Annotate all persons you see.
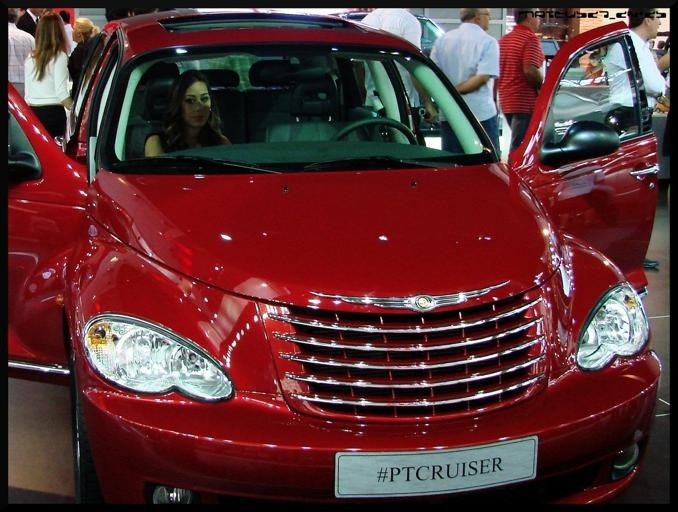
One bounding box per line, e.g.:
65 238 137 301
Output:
657 50 670 225
580 7 671 135
492 7 544 167
426 6 501 159
142 68 232 156
7 9 99 145
359 8 438 148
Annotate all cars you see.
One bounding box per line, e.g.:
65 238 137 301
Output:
332 11 446 56
540 40 563 68
8 7 662 508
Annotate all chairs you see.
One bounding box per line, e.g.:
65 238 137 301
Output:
124 58 361 147
